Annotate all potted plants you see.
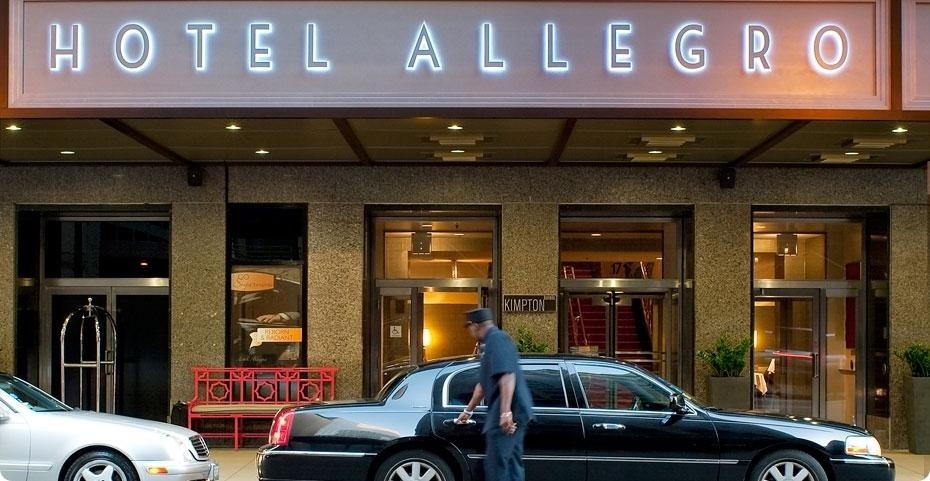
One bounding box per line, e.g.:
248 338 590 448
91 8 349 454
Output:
698 332 755 412
892 341 930 456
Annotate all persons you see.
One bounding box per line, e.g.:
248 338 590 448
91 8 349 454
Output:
455 307 539 481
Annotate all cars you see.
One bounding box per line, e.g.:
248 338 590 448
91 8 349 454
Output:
253 344 901 480
751 346 890 423
0 369 224 480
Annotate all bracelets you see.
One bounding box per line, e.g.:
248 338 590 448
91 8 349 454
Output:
463 408 474 416
499 411 513 418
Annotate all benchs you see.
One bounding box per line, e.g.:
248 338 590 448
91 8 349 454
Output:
187 367 337 453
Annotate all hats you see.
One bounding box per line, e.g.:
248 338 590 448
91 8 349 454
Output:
463 307 493 328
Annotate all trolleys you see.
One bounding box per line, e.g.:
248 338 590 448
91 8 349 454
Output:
58 294 121 415
